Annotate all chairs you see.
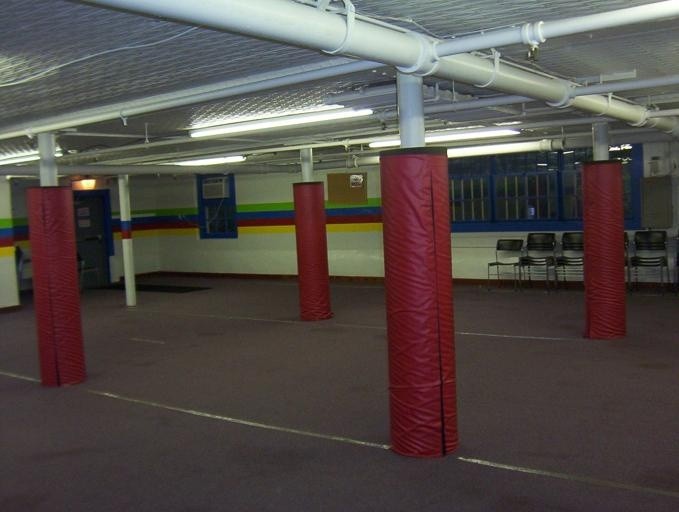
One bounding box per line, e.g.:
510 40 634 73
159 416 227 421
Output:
486 231 672 297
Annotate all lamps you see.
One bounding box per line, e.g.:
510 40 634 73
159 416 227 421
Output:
0 102 521 167
79 175 96 190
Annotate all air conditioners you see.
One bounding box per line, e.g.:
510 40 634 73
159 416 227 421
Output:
203 176 230 199
